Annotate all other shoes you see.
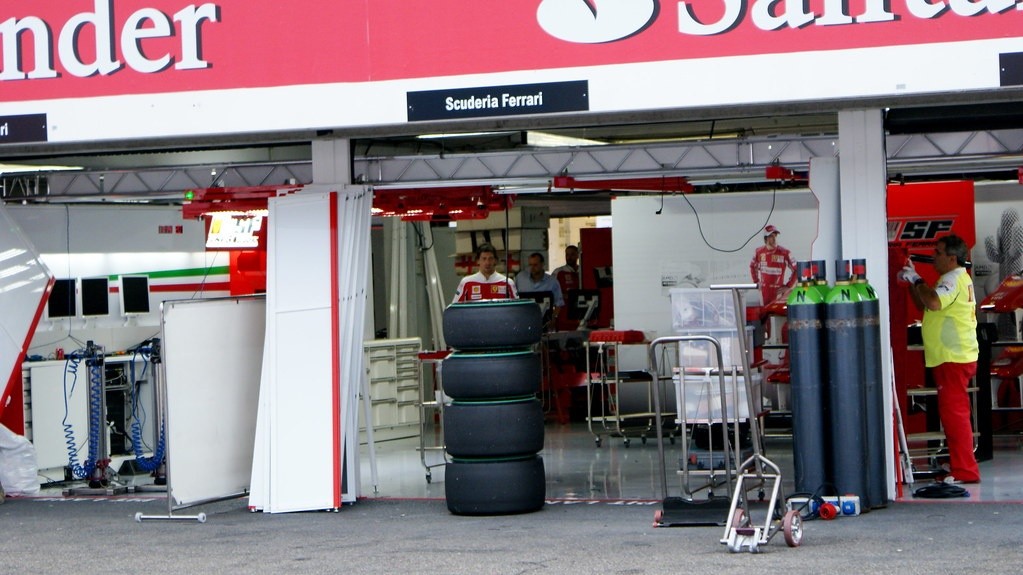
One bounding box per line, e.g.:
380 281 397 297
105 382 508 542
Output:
936 475 948 483
944 475 966 484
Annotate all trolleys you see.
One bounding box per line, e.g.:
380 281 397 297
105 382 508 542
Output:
583 328 678 449
416 350 452 487
708 282 805 553
644 335 775 527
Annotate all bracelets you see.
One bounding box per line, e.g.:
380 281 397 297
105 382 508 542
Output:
914 278 926 288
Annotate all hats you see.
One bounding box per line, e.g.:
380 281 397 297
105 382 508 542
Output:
764 225 780 237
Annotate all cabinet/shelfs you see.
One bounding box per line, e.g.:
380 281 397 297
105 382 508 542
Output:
356 337 425 445
21 354 166 490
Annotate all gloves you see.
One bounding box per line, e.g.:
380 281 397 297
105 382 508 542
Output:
900 259 923 286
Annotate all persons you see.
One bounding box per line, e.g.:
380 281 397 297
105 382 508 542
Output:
452 243 519 302
514 245 565 327
749 224 797 306
902 234 980 483
551 245 579 298
